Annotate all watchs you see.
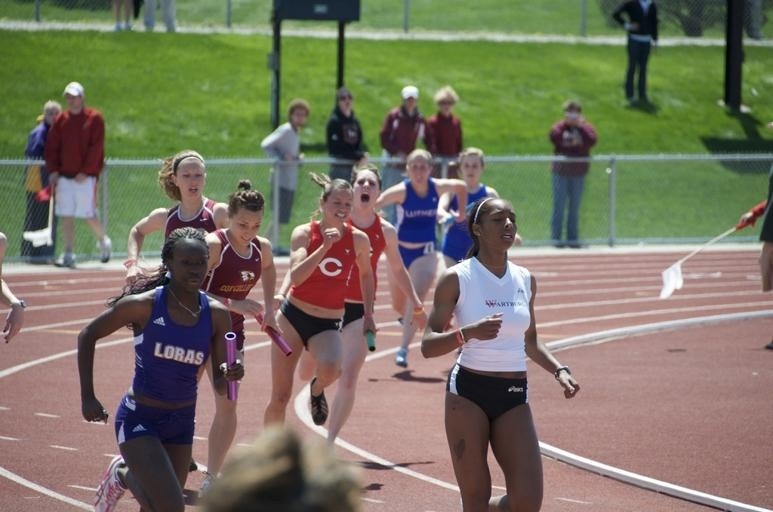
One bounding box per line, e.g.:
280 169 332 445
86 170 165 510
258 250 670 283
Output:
10 300 27 309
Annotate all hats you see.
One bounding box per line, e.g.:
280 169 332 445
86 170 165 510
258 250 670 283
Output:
62 82 84 97
399 86 420 100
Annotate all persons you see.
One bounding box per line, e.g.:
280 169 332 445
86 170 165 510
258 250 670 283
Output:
375 143 472 370
113 2 176 32
260 99 311 257
437 147 524 269
612 0 659 110
74 223 247 511
549 99 596 248
415 194 582 511
24 82 112 269
0 228 31 351
196 177 288 495
260 177 383 433
184 421 376 510
121 142 234 299
735 161 773 354
380 85 464 227
326 87 371 186
268 158 429 450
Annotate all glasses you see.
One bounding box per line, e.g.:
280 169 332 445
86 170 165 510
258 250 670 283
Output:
341 95 353 100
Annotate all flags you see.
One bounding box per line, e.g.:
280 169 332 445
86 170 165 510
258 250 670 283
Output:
658 257 685 301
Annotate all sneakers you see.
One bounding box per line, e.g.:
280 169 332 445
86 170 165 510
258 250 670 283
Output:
97 235 112 262
394 351 406 367
94 455 126 512
309 377 329 426
54 252 78 266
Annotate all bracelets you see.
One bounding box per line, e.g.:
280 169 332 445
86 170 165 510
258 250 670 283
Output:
459 328 466 344
554 365 572 380
362 311 375 321
124 258 138 267
456 330 463 345
412 306 427 316
